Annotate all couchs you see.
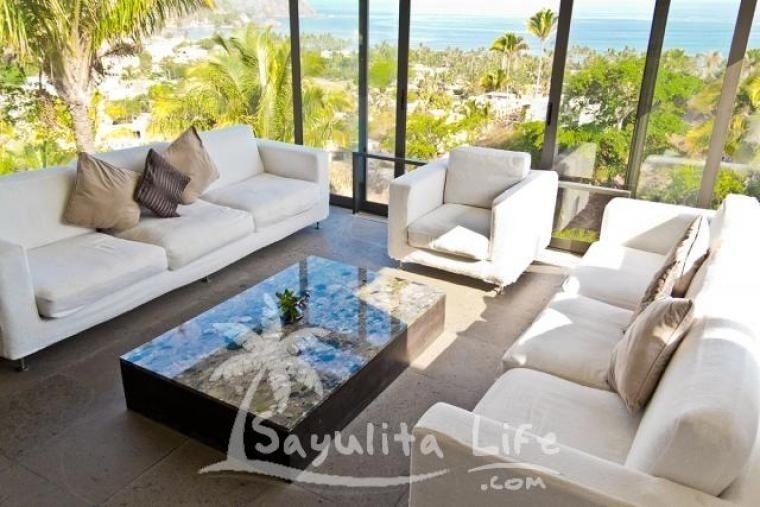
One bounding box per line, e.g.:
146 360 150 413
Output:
1 124 329 370
387 143 559 288
407 192 760 507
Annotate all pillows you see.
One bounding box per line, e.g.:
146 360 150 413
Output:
133 147 192 217
160 125 220 206
622 260 681 335
604 295 695 414
663 213 710 296
61 152 142 229
